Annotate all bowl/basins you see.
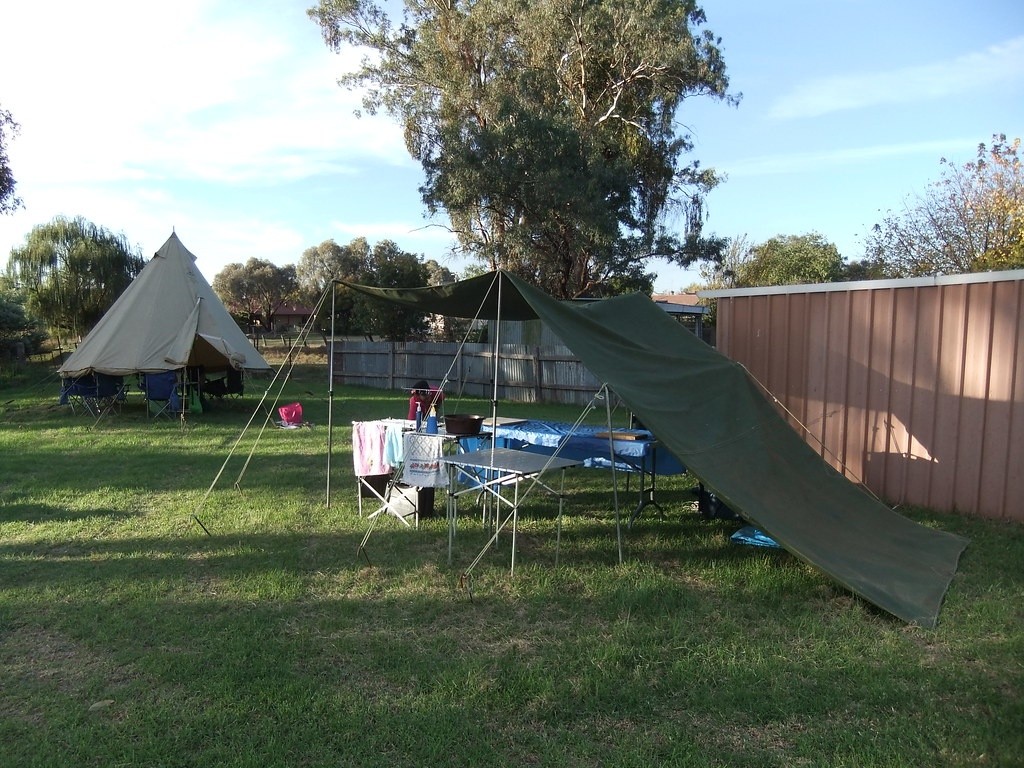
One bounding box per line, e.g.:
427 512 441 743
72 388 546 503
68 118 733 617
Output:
444 413 485 436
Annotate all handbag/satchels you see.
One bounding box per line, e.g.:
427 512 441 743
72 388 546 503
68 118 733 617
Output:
277 402 303 424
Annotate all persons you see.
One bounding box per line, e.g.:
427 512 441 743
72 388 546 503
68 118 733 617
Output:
406 381 445 421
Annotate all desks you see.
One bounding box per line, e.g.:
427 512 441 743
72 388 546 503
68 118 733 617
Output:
351 418 494 532
456 417 666 531
432 447 583 579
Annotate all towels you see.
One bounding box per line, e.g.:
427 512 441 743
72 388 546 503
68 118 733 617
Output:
402 430 451 488
382 420 412 472
350 420 394 477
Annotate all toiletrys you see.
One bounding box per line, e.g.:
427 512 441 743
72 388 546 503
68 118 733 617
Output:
426 402 441 435
413 400 422 434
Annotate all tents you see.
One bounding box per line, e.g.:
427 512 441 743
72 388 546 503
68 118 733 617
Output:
55 226 278 435
202 266 972 632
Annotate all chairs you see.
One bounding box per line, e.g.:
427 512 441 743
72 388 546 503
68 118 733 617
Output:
61 364 246 424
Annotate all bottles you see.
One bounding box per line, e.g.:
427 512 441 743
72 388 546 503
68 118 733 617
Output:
426 403 439 435
415 401 423 433
